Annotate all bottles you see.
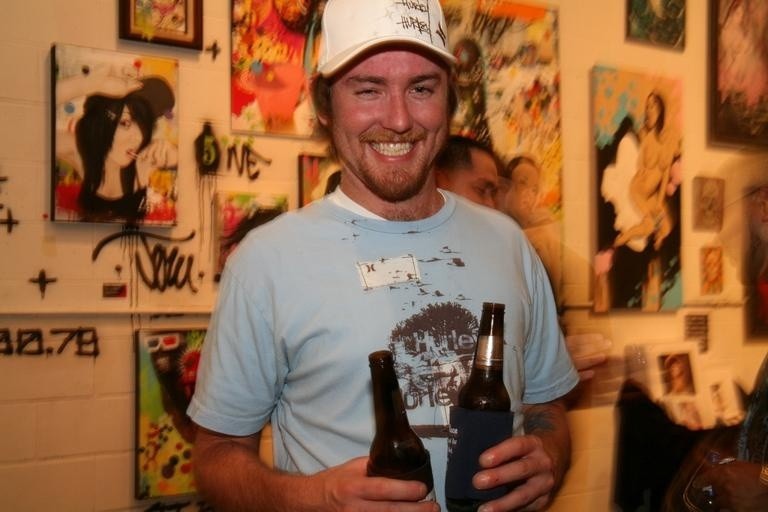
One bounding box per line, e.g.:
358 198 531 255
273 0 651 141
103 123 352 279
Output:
446 302 523 511
368 349 436 507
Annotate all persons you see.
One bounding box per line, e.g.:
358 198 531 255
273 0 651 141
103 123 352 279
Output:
435 136 498 209
56 59 177 228
595 88 676 276
505 155 552 231
187 0 579 512
145 332 188 415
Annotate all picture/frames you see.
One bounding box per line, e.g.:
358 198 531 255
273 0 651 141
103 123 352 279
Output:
119 0 203 50
707 0 768 150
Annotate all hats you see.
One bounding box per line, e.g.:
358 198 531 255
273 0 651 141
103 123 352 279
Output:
316 2 460 78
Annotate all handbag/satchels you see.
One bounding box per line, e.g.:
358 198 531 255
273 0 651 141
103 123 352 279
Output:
661 439 767 511
